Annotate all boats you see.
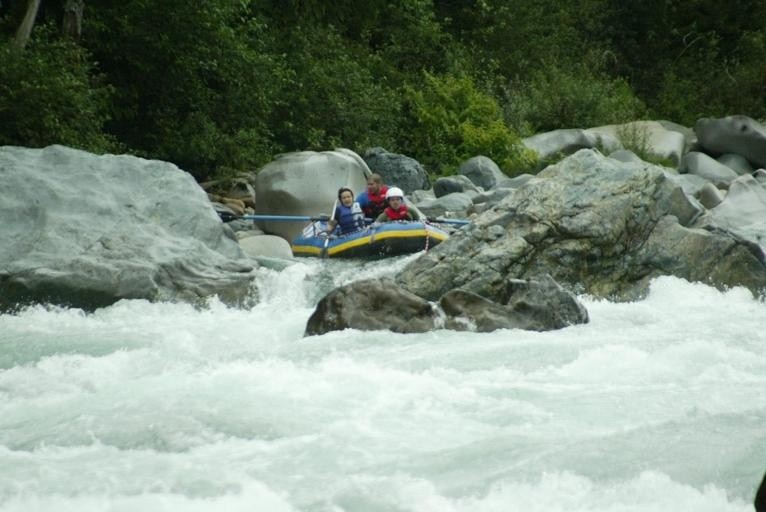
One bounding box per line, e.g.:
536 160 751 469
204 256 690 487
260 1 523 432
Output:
294 212 451 261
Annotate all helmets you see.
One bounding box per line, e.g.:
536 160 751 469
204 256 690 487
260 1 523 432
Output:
384 187 404 202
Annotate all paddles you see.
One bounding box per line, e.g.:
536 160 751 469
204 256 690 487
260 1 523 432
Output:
216 210 330 223
319 198 338 259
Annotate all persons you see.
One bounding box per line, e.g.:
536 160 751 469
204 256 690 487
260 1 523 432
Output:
353 173 391 220
373 185 419 225
326 187 368 238
